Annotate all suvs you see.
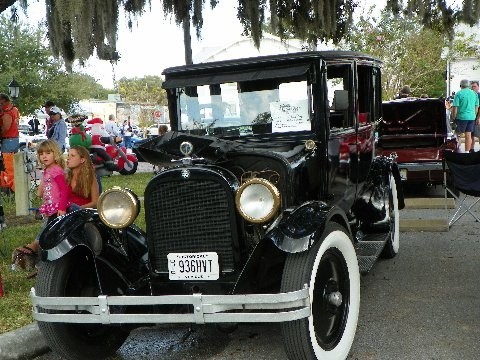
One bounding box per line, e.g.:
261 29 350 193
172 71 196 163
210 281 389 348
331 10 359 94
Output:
384 84 456 185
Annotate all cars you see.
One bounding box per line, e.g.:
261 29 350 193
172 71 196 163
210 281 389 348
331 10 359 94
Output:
28 51 404 360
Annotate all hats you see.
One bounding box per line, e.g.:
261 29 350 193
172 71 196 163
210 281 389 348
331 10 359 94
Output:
43 101 54 107
49 107 61 114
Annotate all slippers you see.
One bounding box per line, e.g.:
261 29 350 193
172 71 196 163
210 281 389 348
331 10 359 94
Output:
16 246 38 255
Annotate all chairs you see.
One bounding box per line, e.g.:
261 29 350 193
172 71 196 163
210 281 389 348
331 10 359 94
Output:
0 152 16 204
441 145 480 229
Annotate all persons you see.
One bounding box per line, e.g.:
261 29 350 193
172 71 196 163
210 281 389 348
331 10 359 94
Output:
43 101 67 154
420 94 429 98
106 114 118 142
181 109 188 126
445 79 480 153
393 84 410 100
38 140 69 217
119 119 132 149
65 112 122 171
28 116 40 135
159 126 168 135
15 145 100 256
0 93 20 154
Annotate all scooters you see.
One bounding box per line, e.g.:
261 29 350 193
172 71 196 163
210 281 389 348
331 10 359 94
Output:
90 136 138 178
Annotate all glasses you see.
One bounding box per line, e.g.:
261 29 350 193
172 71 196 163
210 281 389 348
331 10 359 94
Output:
48 112 56 116
